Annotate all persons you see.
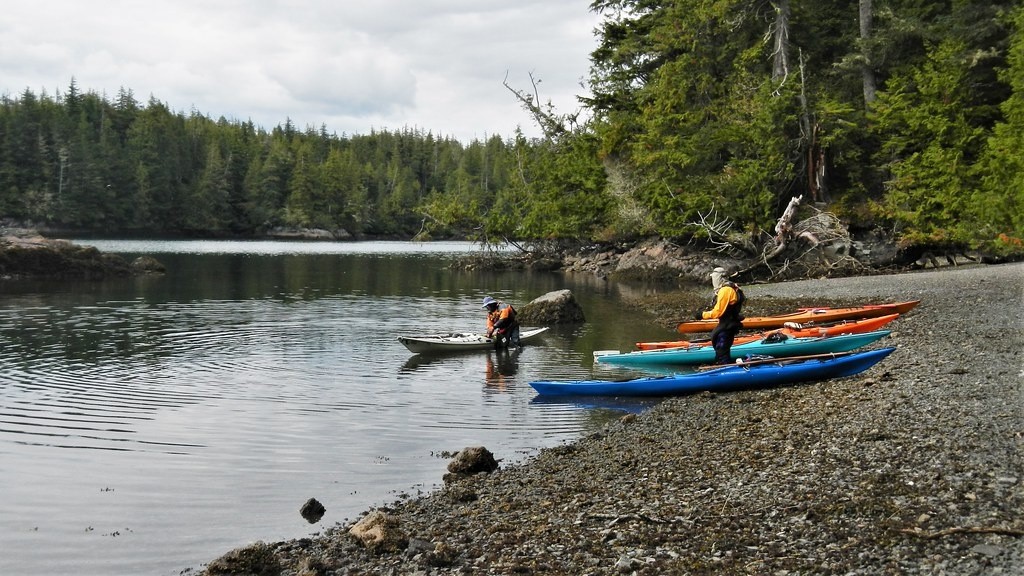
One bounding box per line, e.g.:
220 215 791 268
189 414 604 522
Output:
695 268 745 365
482 296 520 351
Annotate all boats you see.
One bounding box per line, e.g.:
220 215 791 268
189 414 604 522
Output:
677 298 921 336
529 346 898 400
397 327 551 354
636 312 900 350
593 328 891 373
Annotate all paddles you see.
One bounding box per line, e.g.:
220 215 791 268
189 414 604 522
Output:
797 304 896 311
699 351 851 370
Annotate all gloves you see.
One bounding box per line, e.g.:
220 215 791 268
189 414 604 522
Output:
695 312 703 320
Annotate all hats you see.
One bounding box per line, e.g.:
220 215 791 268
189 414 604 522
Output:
482 296 497 308
